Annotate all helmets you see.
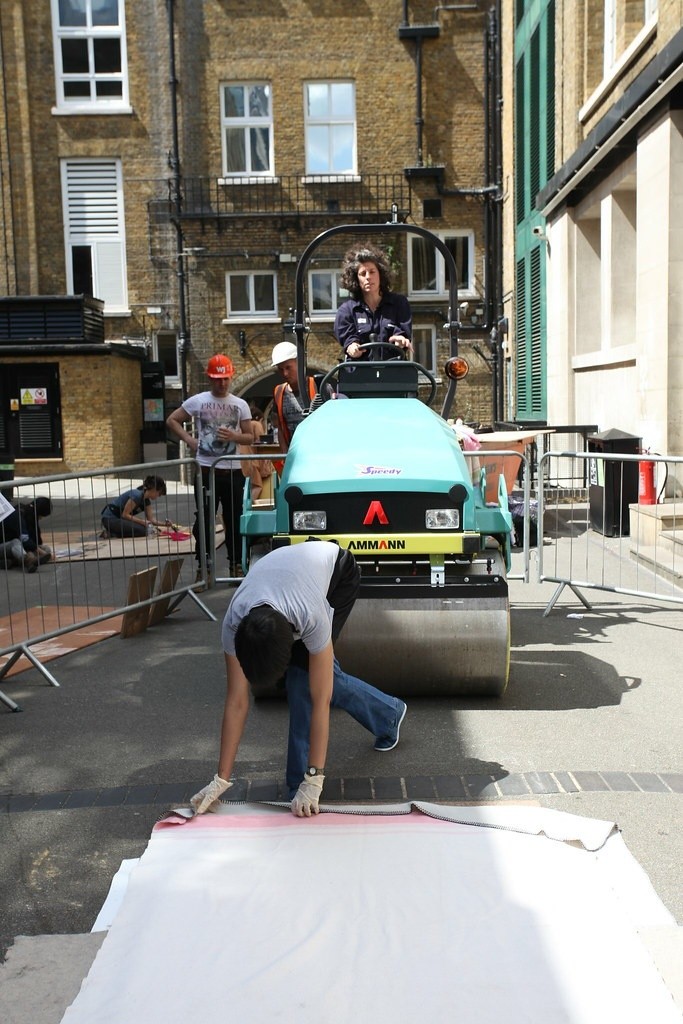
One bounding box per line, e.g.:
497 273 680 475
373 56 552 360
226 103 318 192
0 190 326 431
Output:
207 354 235 379
271 342 297 367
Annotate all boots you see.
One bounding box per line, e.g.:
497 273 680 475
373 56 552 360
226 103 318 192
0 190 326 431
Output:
25 552 52 572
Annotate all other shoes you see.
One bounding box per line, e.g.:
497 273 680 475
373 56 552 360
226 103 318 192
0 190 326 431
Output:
230 566 244 587
99 530 110 539
193 571 210 592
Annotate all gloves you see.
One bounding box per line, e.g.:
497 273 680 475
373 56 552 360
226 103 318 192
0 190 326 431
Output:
291 773 326 817
190 774 233 814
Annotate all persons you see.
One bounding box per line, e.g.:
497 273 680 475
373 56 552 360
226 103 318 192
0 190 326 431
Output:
101 476 171 539
271 341 319 452
166 354 255 590
334 247 414 362
0 497 50 573
190 541 407 819
240 408 265 505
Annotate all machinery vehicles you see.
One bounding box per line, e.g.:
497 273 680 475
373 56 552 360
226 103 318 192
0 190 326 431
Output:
240 221 513 713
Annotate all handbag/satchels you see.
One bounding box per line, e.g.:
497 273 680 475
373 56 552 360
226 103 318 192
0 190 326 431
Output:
259 457 274 478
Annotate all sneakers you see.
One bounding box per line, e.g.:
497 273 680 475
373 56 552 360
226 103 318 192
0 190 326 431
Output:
374 698 407 751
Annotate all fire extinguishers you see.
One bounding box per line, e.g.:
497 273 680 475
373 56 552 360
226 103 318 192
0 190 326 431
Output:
635 446 661 505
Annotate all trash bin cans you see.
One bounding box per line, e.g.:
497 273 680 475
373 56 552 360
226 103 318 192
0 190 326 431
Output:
586 427 643 538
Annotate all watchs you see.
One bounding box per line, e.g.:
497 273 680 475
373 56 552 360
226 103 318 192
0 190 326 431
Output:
306 766 324 776
